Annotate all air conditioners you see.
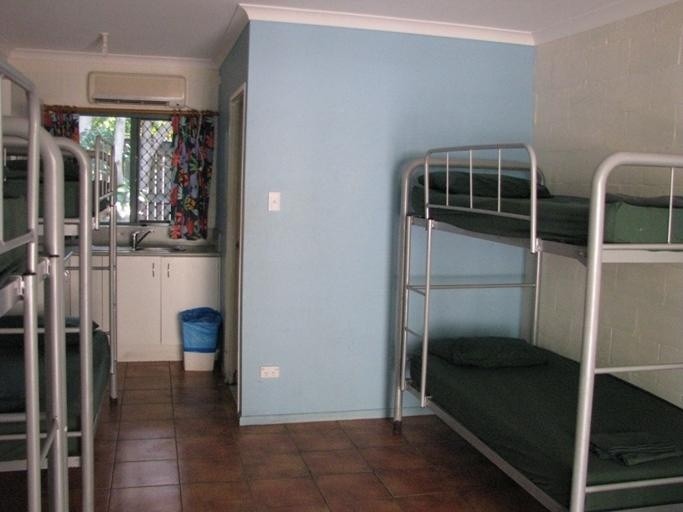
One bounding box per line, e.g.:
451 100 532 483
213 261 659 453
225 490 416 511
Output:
87 71 186 107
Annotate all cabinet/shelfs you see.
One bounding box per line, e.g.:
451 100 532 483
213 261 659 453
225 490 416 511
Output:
5 256 221 362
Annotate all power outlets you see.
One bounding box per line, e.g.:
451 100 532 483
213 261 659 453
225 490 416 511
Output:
259 366 279 379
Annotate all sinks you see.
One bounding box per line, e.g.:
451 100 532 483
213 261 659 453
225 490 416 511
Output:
92 244 132 253
136 246 185 252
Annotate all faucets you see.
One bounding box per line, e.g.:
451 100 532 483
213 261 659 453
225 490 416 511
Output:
131 229 153 251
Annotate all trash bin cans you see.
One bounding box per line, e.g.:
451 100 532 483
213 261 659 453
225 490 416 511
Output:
177 307 222 372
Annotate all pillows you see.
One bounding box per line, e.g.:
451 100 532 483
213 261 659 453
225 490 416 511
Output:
418 170 552 197
422 337 549 369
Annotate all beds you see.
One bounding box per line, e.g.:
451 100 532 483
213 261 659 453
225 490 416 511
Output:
388 143 682 512
